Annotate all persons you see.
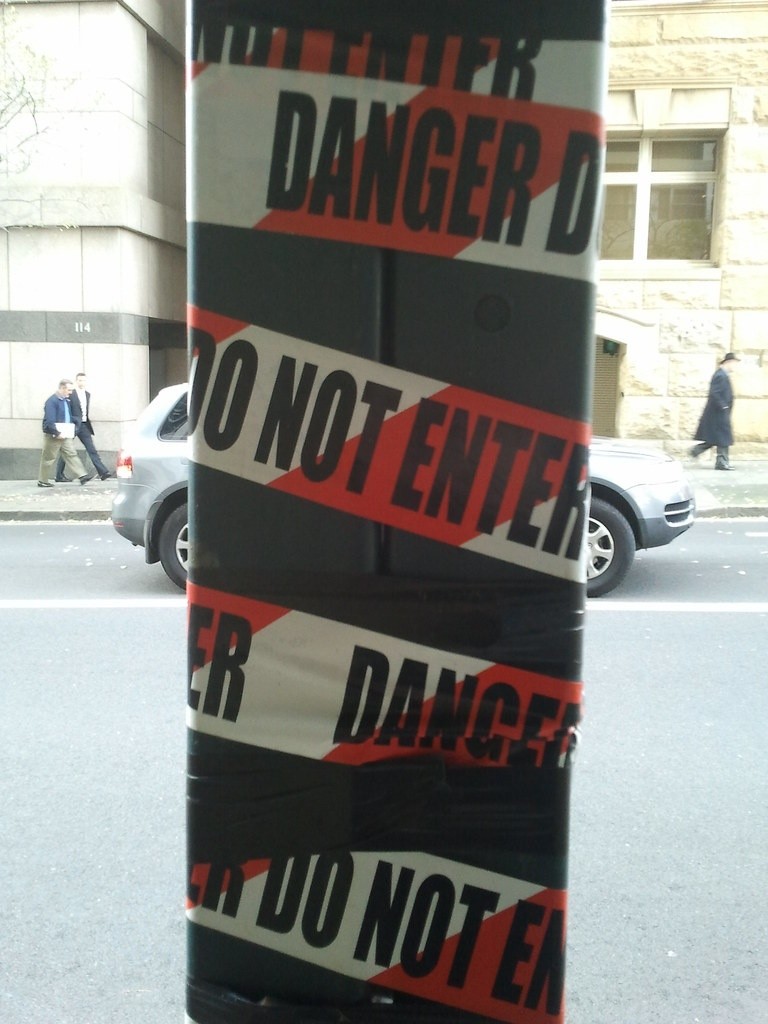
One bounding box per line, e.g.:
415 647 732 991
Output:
55 373 117 482
36 380 98 487
690 352 742 470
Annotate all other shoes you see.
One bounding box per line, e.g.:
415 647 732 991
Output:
55 476 72 482
38 481 53 487
80 476 94 485
715 464 736 470
101 470 116 481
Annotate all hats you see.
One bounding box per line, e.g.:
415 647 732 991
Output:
719 353 741 364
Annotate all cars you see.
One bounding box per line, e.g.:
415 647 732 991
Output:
112 378 696 596
